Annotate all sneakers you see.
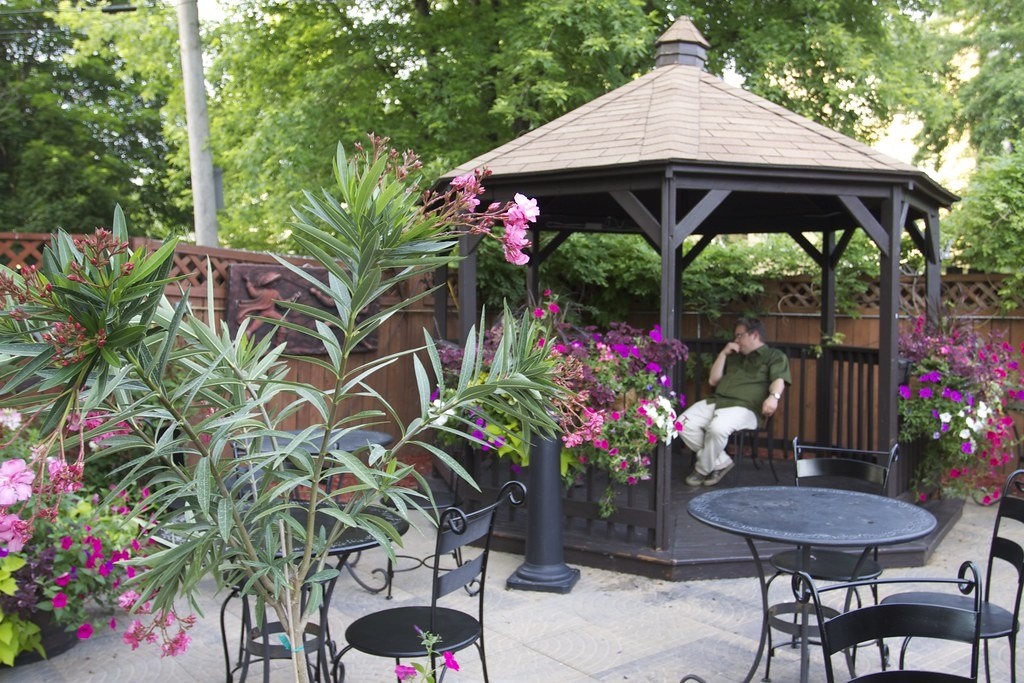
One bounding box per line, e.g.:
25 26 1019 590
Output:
704 463 735 486
686 470 704 486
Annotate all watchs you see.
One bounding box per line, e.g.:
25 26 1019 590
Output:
769 393 781 399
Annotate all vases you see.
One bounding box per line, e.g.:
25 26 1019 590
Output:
6 594 80 667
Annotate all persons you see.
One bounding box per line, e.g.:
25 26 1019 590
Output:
676 317 791 486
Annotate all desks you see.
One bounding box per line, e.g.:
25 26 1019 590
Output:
197 496 410 683
234 427 393 504
679 485 936 683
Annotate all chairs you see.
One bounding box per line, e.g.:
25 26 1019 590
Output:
689 379 781 483
380 403 481 600
234 442 339 509
765 437 1024 683
333 481 531 683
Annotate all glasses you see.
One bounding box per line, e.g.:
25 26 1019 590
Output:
736 331 749 337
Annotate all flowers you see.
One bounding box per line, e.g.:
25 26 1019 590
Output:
0 408 194 670
